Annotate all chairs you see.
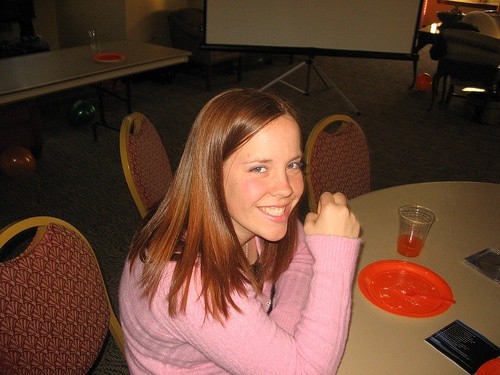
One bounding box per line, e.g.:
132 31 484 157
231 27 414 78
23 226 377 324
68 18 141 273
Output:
440 29 500 102
120 111 173 219
168 8 242 91
303 114 370 215
6 34 74 157
0 216 130 375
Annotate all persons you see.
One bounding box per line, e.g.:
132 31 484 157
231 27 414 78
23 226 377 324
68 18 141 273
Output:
120 88 362 375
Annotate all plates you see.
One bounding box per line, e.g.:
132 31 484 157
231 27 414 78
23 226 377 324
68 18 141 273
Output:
358 259 454 318
90 52 127 63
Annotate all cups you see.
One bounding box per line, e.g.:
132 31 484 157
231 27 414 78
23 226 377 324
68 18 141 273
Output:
396 205 435 258
87 30 100 56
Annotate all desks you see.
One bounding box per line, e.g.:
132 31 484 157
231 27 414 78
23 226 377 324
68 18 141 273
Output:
336 182 500 375
0 40 190 142
408 23 447 94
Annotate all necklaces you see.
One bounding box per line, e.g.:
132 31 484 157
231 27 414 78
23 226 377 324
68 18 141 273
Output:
245 242 248 259
